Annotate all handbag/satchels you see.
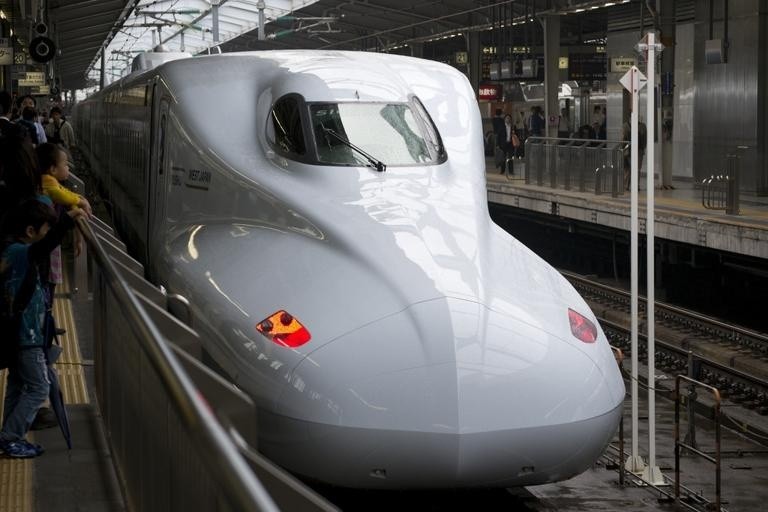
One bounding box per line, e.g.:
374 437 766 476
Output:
511 135 520 147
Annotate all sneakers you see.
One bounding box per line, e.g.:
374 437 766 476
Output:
0 438 45 458
28 407 59 430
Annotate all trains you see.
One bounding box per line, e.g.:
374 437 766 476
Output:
66 43 628 497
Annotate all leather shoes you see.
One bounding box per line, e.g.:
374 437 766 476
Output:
54 328 65 335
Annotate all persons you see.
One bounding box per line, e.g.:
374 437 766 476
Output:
0 86 92 460
491 104 646 194
